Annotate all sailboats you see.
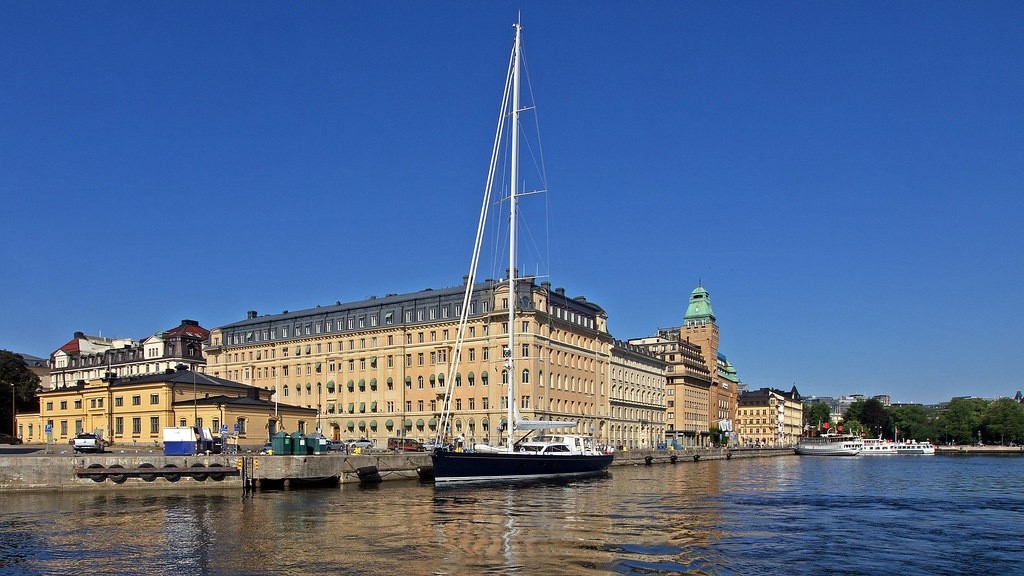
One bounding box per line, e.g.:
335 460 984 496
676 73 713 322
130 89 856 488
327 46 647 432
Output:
427 7 612 486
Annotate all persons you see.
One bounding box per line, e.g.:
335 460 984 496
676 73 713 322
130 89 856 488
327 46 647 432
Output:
458 434 465 452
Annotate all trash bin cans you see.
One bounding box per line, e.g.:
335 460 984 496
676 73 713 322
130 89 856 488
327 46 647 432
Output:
304 432 328 454
271 431 292 455
162 426 204 455
290 431 308 454
197 426 214 453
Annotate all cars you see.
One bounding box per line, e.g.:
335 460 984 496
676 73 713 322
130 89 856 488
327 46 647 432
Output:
423 442 440 451
326 440 345 453
345 439 374 449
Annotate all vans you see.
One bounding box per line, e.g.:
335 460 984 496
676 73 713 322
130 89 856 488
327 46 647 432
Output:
387 438 423 452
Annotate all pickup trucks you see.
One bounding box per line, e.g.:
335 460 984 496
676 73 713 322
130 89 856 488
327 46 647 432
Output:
69 432 106 453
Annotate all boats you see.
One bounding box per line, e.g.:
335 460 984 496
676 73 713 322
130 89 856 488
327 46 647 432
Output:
859 437 935 455
787 430 862 455
